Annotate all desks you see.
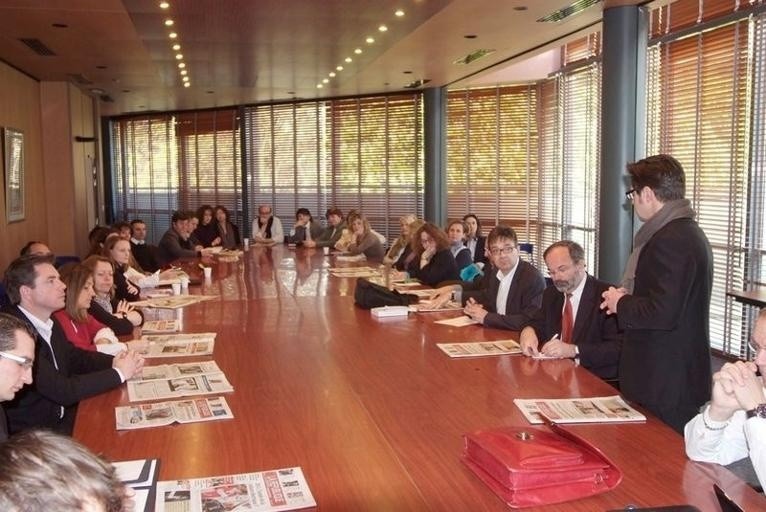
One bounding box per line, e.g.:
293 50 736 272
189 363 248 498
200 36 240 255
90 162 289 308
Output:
71 244 766 512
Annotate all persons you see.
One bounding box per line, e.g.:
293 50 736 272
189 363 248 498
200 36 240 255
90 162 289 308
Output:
601 154 714 437
684 305 766 498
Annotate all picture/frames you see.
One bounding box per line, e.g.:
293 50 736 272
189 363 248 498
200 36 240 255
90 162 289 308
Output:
2 126 25 225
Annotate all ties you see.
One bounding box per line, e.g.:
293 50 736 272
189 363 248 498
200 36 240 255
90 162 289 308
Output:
561 293 574 345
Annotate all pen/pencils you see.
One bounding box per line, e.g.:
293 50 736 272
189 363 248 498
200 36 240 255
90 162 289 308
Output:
541 333 559 355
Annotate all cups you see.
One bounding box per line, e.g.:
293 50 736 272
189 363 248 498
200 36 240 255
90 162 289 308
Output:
171 266 213 295
323 246 330 255
243 237 251 246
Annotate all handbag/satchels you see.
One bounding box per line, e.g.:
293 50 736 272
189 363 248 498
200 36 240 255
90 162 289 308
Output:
459 410 624 509
353 277 420 310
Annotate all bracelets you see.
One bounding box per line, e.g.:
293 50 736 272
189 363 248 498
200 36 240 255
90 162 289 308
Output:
701 402 731 432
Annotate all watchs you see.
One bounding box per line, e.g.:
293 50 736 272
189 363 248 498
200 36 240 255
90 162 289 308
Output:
740 404 765 419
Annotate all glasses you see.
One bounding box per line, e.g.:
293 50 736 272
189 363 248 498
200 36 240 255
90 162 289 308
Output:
748 338 766 353
0 350 33 370
625 187 638 200
490 246 514 256
